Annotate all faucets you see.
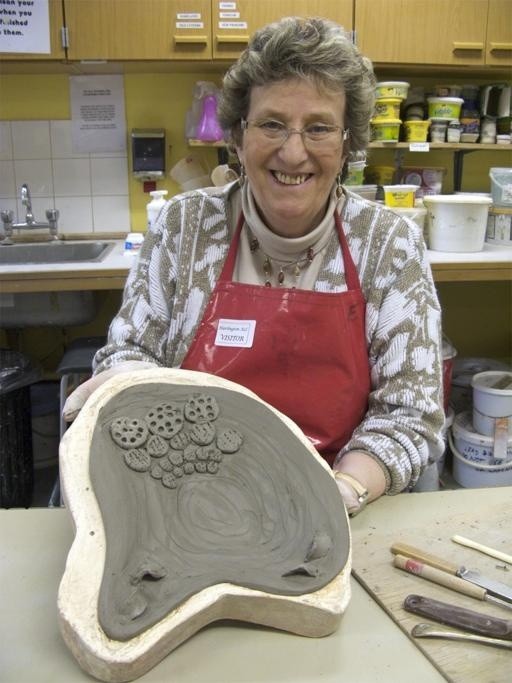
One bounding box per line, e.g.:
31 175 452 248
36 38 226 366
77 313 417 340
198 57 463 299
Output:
21 182 34 223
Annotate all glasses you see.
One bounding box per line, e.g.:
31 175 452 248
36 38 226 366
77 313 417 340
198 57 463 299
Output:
238 115 350 152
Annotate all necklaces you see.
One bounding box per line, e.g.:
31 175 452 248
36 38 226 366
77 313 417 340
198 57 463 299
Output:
246 232 315 289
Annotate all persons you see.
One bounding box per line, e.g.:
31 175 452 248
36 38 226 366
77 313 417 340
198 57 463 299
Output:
60 17 447 515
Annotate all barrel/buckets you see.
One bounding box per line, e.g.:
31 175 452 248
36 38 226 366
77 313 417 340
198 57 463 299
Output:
489 167 512 206
438 339 458 417
448 355 512 417
471 370 512 438
449 411 512 489
390 207 427 231
423 195 495 252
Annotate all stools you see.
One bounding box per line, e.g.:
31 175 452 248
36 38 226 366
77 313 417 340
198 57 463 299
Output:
49 337 108 512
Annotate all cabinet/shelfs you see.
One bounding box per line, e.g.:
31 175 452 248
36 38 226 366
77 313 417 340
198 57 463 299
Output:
63 0 354 62
354 0 512 72
0 0 64 63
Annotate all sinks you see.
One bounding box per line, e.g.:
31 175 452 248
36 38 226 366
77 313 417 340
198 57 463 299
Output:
0 240 116 263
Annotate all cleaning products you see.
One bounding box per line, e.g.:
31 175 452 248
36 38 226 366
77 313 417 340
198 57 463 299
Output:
147 191 168 225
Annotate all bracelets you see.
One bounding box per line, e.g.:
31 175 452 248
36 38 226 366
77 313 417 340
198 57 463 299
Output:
335 470 369 516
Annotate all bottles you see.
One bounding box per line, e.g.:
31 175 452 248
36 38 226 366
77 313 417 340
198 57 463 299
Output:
125 232 145 256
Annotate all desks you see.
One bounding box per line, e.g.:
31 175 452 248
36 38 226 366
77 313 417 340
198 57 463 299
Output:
0 484 512 682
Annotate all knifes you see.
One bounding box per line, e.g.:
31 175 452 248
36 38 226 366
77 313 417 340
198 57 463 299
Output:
389 544 512 603
